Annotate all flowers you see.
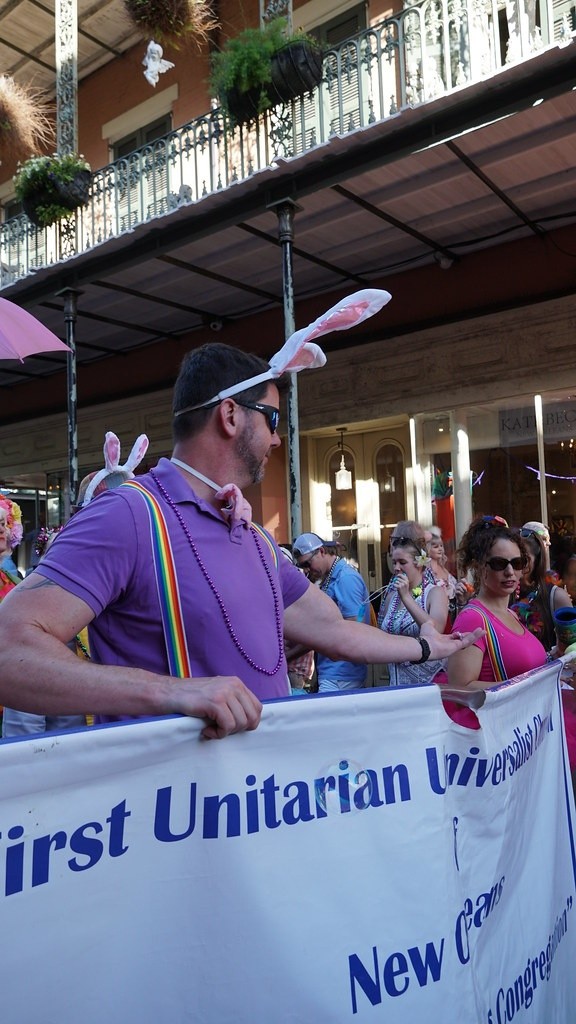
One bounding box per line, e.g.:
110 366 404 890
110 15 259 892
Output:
11 152 92 206
0 494 24 548
412 586 423 599
413 548 433 568
34 525 64 556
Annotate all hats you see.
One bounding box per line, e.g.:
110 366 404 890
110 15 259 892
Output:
71 471 131 508
293 532 336 557
520 521 549 544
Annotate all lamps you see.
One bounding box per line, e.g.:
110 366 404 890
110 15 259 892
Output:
379 449 396 493
334 427 353 490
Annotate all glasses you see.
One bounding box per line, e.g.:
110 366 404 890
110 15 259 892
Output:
393 538 421 555
296 551 316 568
205 398 280 434
519 529 540 546
481 556 525 571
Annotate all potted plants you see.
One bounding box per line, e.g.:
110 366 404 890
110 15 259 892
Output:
202 19 327 127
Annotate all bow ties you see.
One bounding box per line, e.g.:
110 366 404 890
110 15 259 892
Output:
171 458 252 531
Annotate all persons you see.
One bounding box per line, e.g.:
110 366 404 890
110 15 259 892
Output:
277 531 377 694
376 516 576 772
0 467 149 738
0 342 490 739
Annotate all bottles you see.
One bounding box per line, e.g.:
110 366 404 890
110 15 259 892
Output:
464 569 474 600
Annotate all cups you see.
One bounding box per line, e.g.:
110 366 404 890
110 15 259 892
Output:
554 607 576 650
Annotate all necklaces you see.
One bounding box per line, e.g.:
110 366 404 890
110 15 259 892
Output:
151 470 283 675
324 555 340 593
385 580 426 634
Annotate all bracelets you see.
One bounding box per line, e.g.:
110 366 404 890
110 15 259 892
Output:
409 636 431 664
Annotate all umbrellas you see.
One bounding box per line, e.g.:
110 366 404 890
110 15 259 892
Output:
0 297 74 364
